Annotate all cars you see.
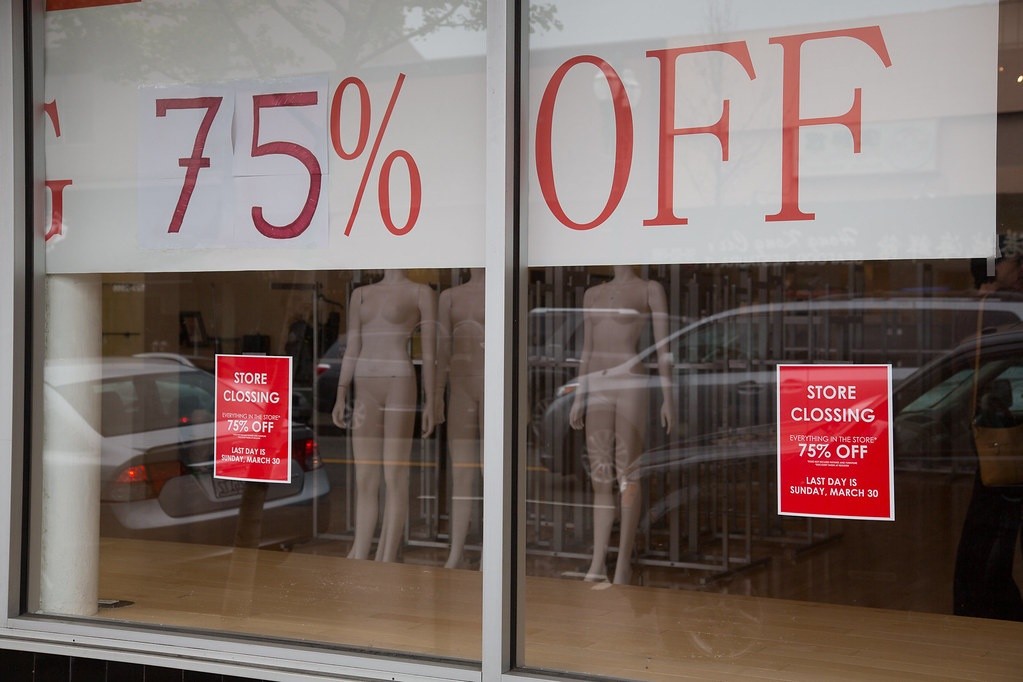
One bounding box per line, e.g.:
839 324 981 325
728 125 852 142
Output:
606 326 1023 663
43 353 331 551
316 319 538 438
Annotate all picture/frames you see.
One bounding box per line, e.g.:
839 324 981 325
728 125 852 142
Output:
177 312 207 348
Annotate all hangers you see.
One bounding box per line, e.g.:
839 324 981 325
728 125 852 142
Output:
317 284 344 310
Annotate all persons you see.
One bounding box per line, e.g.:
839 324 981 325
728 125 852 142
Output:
331 269 438 564
569 265 677 584
953 235 1023 622
434 268 485 571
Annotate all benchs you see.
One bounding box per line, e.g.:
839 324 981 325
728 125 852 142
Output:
101 392 203 437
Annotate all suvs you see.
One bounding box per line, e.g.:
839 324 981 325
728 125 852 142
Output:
538 284 1023 492
